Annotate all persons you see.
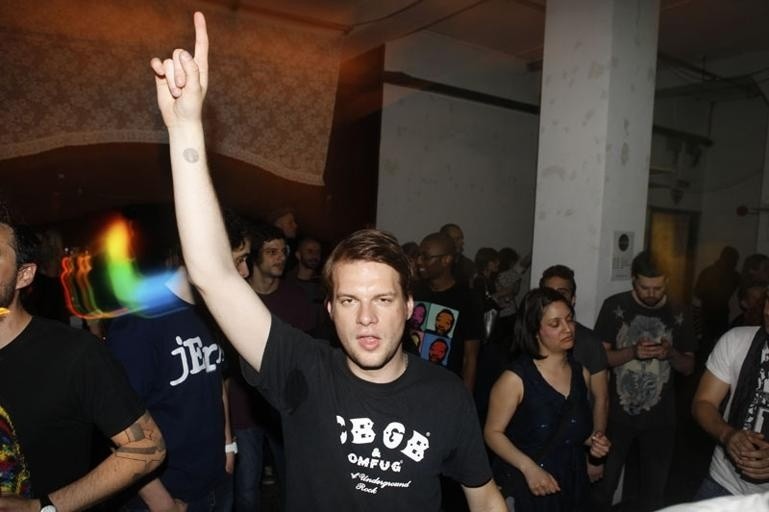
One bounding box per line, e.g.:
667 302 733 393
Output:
1 206 324 510
484 251 696 512
690 254 768 500
403 223 531 426
151 11 511 512
